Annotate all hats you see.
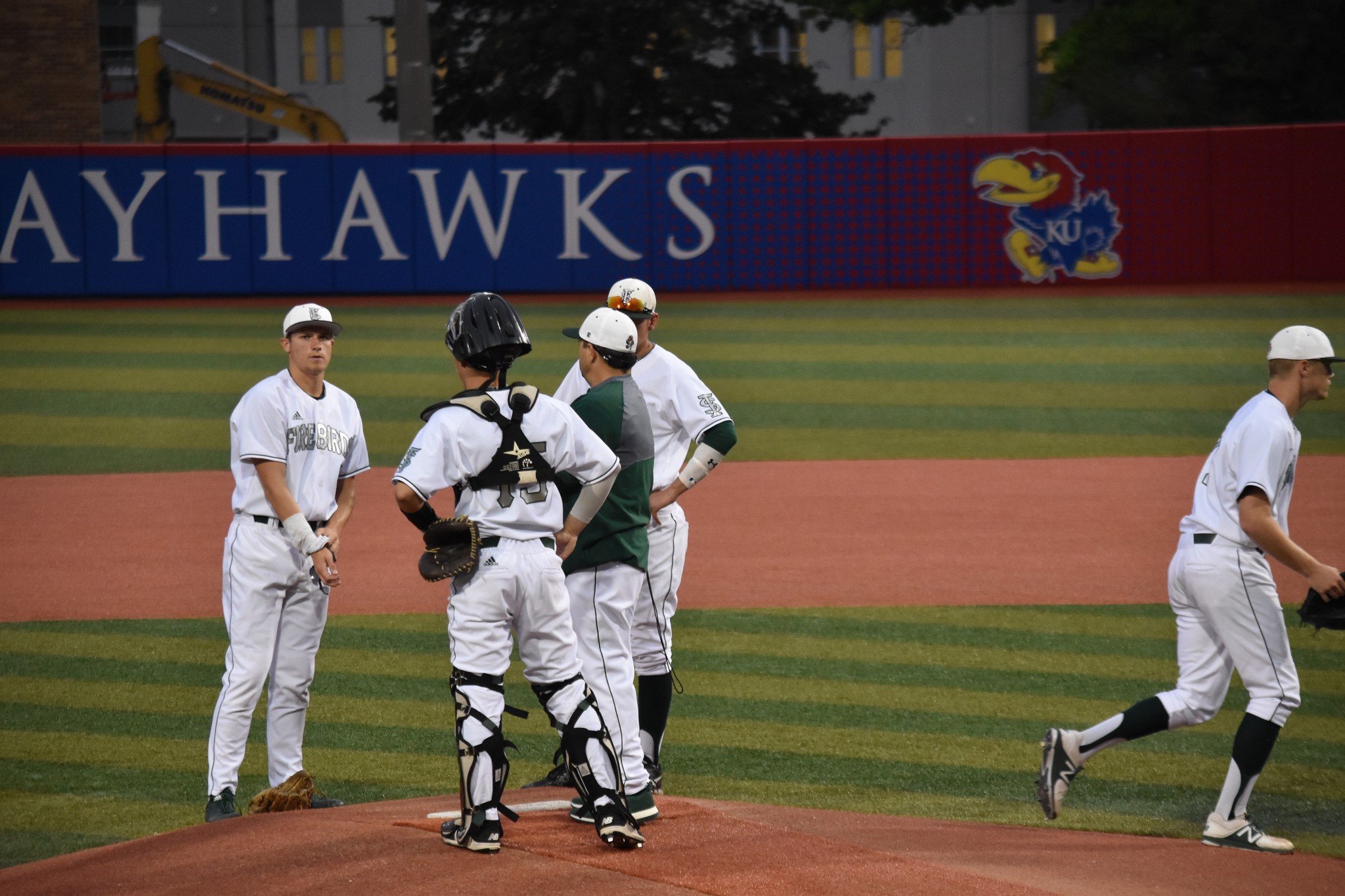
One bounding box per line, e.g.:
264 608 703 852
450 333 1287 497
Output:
563 308 637 353
608 279 656 318
282 303 343 338
1267 325 1345 362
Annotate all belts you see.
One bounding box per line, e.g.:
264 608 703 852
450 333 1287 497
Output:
480 534 556 548
1192 533 1264 555
253 514 329 532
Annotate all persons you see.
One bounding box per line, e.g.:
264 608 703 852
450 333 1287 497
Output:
205 303 372 823
1035 324 1345 853
390 277 737 853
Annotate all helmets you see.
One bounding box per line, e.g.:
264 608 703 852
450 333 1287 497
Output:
445 293 532 369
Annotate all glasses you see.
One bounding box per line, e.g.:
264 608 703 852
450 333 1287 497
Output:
608 296 654 315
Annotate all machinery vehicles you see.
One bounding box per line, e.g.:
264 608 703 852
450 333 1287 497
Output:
134 35 349 145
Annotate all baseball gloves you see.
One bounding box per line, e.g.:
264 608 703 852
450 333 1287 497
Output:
1295 571 1345 633
418 518 480 583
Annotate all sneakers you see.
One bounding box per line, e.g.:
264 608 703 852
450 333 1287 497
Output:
647 765 662 793
1202 812 1295 853
1036 728 1082 819
594 805 646 850
308 791 342 808
572 797 586 809
522 763 572 789
204 787 242 823
570 781 659 825
440 812 503 853
